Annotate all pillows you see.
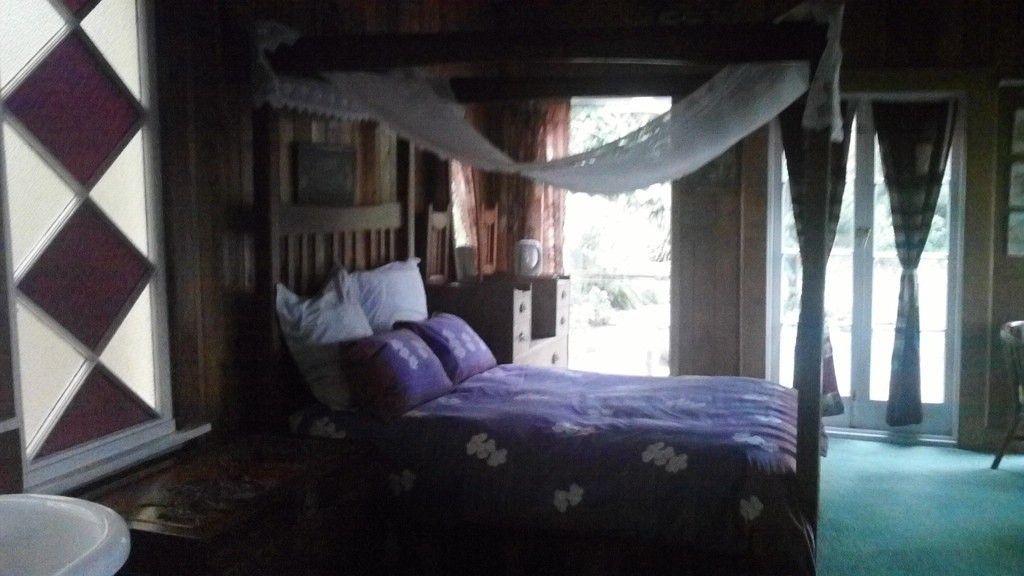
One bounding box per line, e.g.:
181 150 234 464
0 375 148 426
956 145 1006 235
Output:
391 310 495 385
350 257 428 332
275 267 372 412
335 325 455 425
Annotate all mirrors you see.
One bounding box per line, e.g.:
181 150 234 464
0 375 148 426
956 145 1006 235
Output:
445 156 485 284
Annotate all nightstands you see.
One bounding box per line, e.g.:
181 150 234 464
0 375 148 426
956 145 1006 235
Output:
89 434 375 575
513 337 571 369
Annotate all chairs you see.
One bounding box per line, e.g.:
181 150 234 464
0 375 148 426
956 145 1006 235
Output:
990 321 1023 469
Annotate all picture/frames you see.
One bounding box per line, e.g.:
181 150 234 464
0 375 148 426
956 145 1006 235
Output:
1006 104 1024 261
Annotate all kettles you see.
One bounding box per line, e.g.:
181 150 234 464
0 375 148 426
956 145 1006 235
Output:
513 238 543 277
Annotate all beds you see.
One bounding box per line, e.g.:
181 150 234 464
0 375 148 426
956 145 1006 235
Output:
239 1 848 576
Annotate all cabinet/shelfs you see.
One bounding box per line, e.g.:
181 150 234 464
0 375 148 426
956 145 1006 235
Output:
426 275 572 363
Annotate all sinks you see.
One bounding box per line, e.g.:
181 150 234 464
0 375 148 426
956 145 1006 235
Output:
0 494 132 576
